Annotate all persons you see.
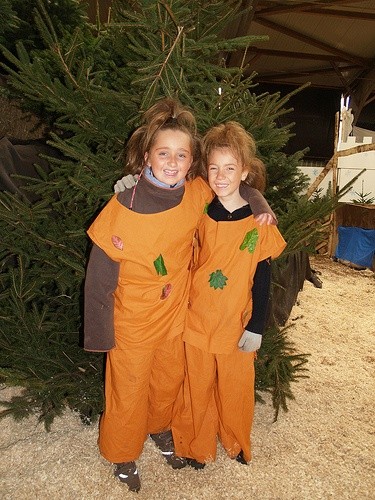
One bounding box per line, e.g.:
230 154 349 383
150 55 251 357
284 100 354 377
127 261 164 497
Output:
112 122 289 472
85 100 276 492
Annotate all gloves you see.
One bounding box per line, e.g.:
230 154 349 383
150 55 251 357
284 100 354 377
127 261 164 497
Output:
113 173 140 194
237 331 262 353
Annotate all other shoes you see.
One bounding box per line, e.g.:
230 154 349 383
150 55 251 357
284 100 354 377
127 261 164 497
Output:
235 451 250 465
188 456 207 470
114 462 143 493
164 451 186 469
